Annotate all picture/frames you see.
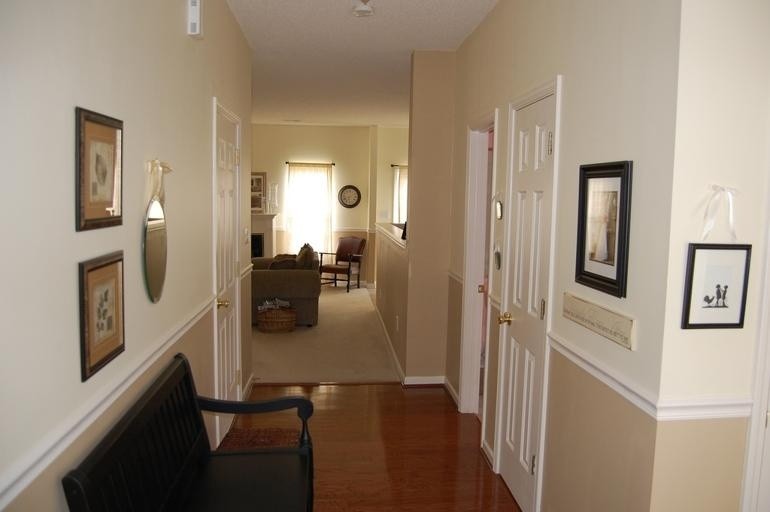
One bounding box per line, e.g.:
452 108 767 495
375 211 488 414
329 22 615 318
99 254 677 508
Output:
251 172 267 213
681 243 752 330
75 105 124 232
79 250 126 384
573 160 633 299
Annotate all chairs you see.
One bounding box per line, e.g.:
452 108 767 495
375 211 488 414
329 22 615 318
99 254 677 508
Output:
319 235 365 292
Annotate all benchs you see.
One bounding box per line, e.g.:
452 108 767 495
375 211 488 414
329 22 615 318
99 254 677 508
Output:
62 352 315 511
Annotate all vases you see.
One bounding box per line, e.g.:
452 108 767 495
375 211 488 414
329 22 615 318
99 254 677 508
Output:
268 182 280 215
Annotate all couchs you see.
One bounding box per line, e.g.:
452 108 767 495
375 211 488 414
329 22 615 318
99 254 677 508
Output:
252 250 320 327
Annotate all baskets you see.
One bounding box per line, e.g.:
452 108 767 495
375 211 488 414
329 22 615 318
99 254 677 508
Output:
256 307 297 333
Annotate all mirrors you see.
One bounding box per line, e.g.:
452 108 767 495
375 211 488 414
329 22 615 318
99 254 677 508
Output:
140 158 173 307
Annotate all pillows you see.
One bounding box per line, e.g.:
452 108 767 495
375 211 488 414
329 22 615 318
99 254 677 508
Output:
273 254 297 259
295 245 313 270
268 258 295 269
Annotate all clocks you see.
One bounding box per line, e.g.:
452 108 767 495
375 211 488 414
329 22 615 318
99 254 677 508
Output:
338 185 361 208
